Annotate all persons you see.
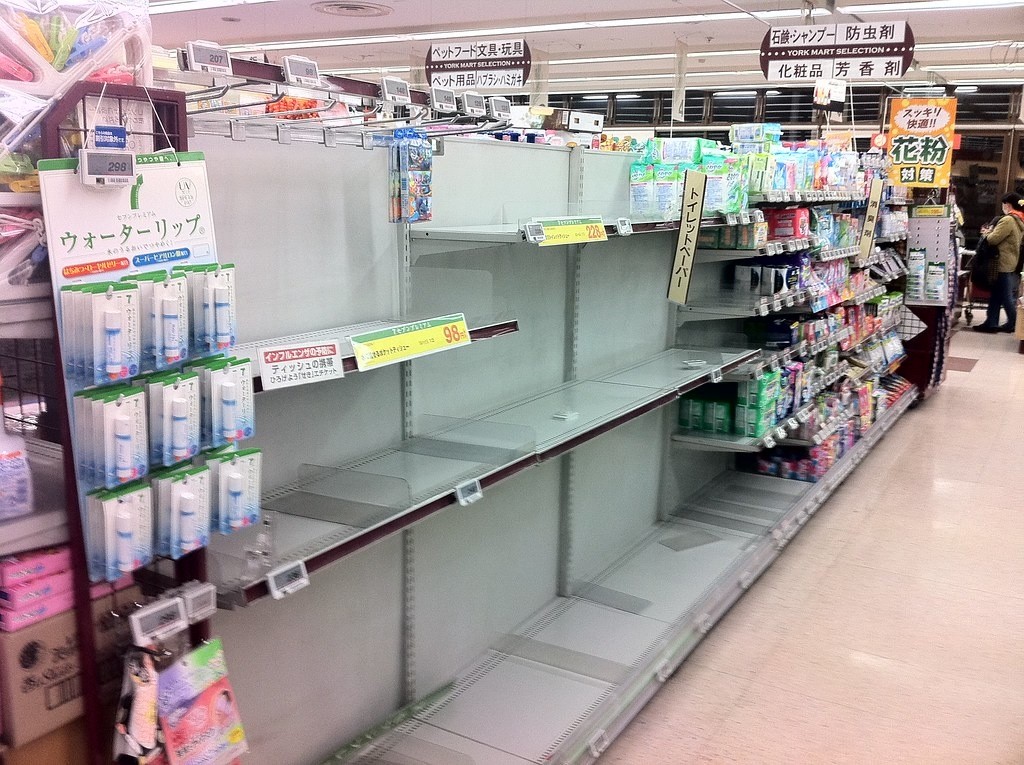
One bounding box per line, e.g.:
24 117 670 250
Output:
971 190 1024 333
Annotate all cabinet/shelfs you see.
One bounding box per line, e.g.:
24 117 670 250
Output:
658 185 921 553
187 116 765 765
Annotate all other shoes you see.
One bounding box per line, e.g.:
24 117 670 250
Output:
973 324 998 333
998 323 1015 333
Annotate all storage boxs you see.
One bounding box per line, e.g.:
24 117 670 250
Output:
0 583 140 750
0 543 133 633
